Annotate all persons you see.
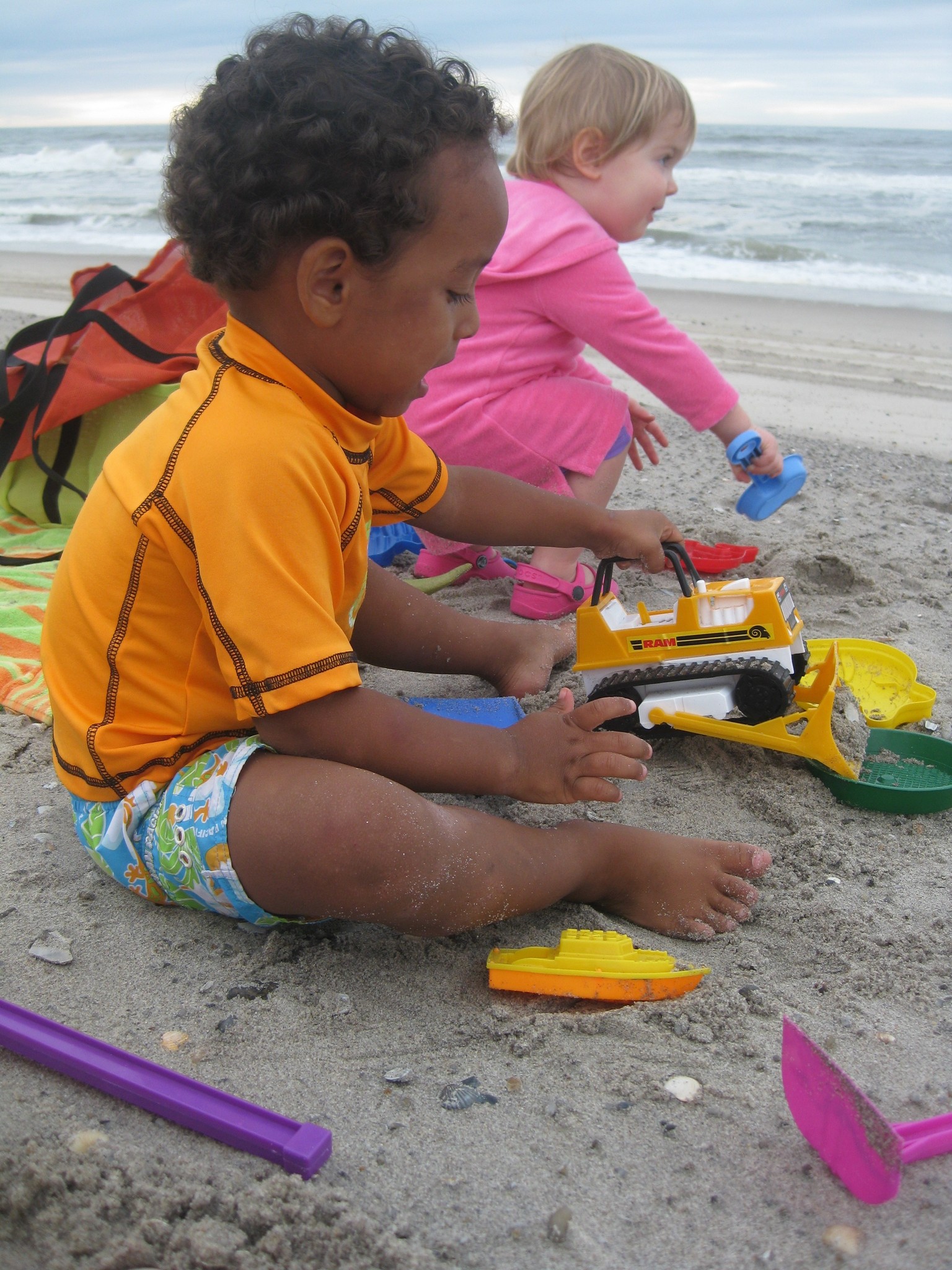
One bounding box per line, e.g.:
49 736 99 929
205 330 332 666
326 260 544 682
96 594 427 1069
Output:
390 46 779 623
41 11 776 944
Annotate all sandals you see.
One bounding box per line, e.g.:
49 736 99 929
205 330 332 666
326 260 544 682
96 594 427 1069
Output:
510 560 619 620
414 543 516 580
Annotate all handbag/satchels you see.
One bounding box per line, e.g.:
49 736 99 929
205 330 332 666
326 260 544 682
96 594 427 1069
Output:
0 236 228 527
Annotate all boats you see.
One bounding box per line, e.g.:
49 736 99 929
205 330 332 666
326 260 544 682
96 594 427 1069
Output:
486 928 709 1005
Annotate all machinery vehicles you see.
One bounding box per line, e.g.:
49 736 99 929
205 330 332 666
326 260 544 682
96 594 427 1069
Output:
570 541 870 782
